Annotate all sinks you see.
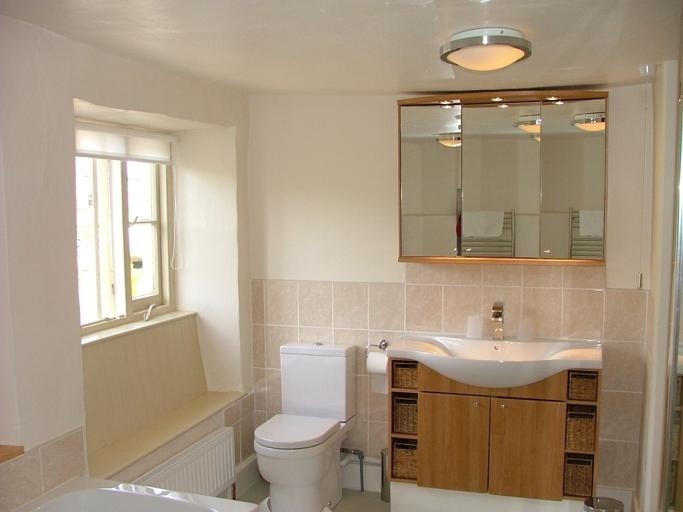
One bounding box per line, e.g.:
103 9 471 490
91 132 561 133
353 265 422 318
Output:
386 330 604 388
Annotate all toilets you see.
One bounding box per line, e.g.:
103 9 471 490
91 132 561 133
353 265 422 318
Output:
253 343 357 512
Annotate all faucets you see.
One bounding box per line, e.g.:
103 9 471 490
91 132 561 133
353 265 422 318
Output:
491 300 505 339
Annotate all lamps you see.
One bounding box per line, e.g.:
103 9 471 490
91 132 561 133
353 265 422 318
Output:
438 27 533 72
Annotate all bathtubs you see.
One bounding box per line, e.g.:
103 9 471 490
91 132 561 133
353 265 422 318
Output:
12 477 260 511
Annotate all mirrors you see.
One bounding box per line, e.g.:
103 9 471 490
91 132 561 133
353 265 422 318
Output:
396 90 610 269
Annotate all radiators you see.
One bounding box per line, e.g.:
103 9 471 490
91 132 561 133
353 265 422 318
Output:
128 426 236 498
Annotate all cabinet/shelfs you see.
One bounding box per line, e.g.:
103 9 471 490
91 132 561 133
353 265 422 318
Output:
385 357 603 512
